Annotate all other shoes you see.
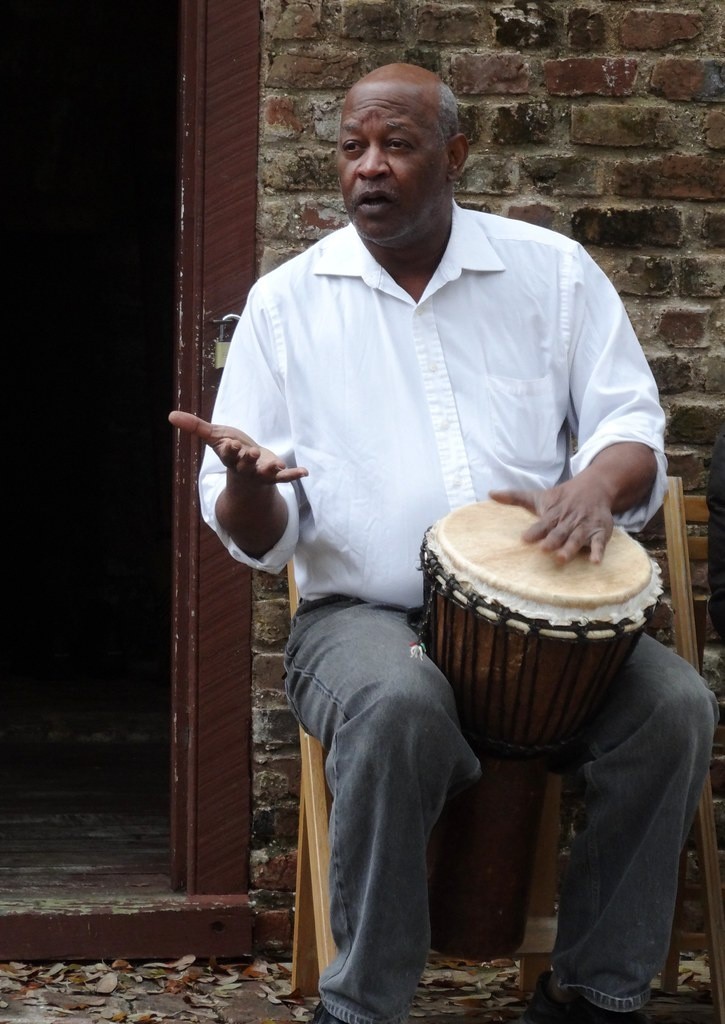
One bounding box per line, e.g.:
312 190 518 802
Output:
312 1001 346 1024
518 968 651 1024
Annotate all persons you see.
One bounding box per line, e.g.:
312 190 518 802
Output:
168 64 720 1024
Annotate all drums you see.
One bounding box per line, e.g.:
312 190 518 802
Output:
416 493 666 962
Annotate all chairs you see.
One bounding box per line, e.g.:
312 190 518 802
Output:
291 476 725 1024
